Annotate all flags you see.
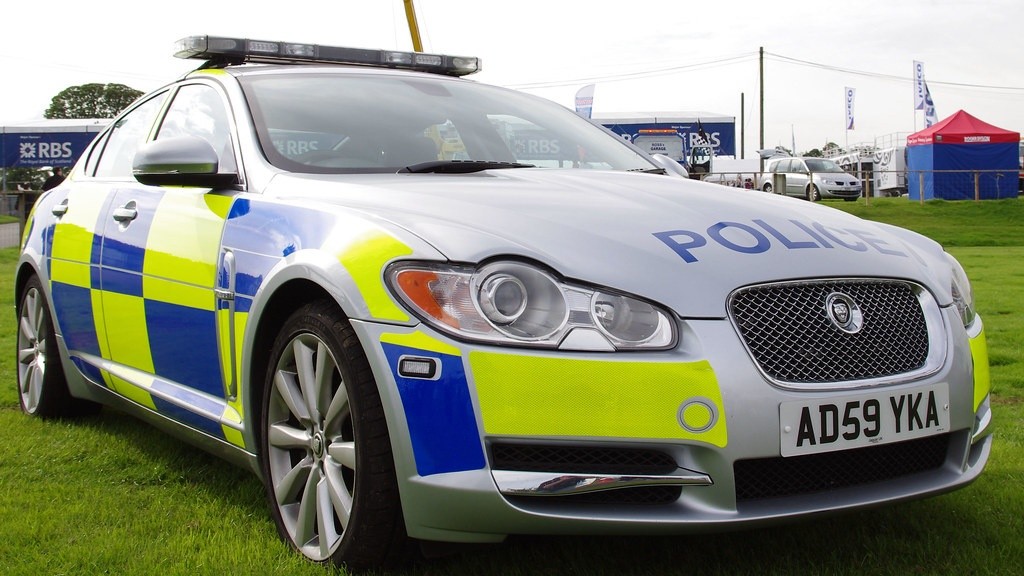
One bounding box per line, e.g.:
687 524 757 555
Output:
699 123 709 143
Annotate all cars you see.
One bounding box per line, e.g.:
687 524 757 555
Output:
13 35 994 576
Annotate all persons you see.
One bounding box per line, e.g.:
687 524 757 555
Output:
42 167 66 190
745 178 754 190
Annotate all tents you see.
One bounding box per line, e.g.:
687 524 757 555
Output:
906 109 1020 200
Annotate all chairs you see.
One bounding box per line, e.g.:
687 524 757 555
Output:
383 136 438 166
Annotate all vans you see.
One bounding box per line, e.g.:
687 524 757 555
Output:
756 149 862 201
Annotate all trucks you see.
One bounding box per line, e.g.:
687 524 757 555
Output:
631 130 712 181
